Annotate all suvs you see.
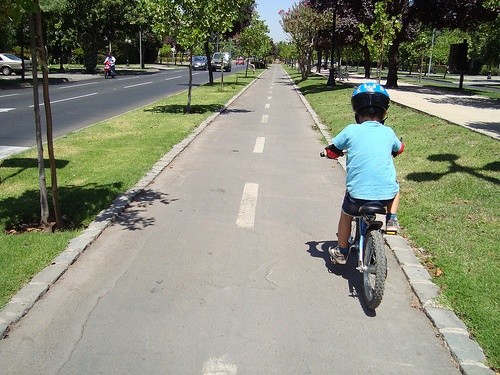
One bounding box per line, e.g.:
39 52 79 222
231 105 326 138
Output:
211 52 232 72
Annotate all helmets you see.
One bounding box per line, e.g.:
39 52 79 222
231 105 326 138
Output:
352 82 390 109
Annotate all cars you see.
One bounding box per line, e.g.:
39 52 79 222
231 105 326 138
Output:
236 58 244 65
0 52 33 76
188 56 209 71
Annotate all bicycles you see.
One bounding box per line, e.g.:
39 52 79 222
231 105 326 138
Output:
104 61 117 79
319 150 398 310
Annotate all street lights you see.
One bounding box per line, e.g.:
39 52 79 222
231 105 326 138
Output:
124 38 132 66
245 36 253 78
55 30 64 71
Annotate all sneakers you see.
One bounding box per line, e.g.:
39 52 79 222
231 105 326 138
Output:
328 244 346 264
386 217 399 231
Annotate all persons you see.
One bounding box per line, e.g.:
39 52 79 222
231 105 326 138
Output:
105 52 116 71
323 81 404 264
